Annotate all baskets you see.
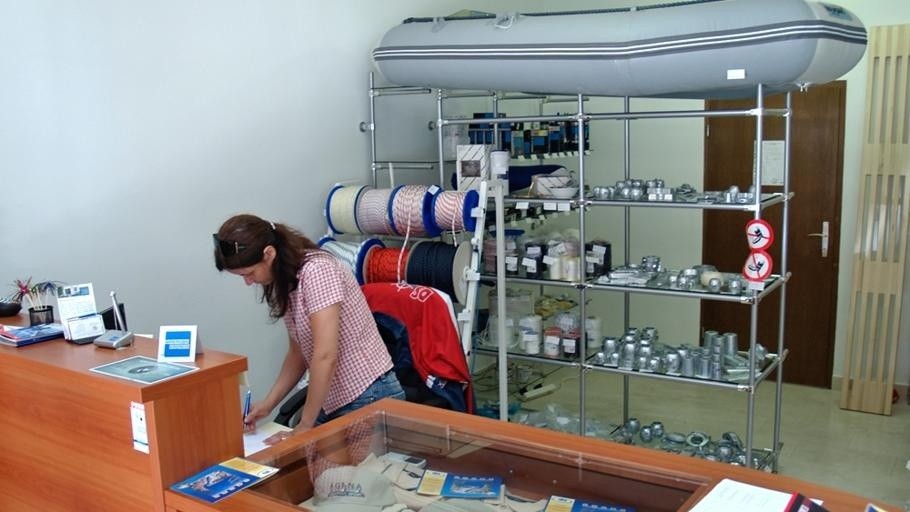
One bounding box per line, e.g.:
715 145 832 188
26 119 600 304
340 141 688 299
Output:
29 306 54 326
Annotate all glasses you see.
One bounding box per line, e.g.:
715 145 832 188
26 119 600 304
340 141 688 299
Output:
213 234 246 258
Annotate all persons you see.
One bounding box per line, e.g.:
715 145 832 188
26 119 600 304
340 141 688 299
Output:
212 215 408 446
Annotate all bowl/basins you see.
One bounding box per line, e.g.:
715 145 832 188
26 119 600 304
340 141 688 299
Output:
0 302 22 318
549 187 578 199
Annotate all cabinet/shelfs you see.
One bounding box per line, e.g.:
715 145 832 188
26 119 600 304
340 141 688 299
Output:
438 84 792 475
166 395 910 512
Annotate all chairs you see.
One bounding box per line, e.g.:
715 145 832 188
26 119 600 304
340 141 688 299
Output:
274 282 476 430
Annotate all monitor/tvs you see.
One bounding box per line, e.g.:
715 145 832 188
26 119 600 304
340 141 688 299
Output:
99 302 127 332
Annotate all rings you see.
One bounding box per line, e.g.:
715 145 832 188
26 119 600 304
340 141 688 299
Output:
281 434 288 440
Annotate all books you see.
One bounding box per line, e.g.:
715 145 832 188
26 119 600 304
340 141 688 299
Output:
0 321 64 348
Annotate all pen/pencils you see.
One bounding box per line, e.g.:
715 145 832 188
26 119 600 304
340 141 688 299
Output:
244 391 252 432
26 287 54 325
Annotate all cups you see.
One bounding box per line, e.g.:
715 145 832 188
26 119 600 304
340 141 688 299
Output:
738 192 754 203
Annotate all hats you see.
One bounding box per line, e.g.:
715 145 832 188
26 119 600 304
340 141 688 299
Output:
313 466 396 512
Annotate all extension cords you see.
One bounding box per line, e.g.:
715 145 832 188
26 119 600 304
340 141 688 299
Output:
522 383 556 402
